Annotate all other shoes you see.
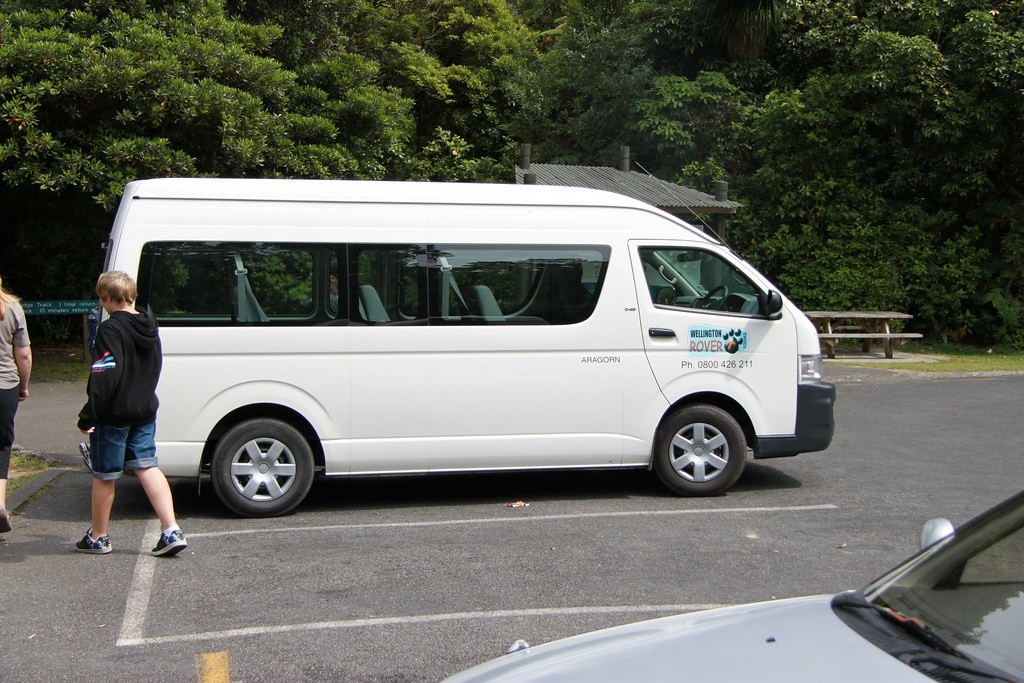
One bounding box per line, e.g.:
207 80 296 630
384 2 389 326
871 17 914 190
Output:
0 517 12 533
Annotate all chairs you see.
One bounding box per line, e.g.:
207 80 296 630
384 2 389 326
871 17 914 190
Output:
359 285 390 322
233 286 260 322
456 285 504 324
548 263 594 324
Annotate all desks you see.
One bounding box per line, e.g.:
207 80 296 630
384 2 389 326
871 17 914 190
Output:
803 312 914 358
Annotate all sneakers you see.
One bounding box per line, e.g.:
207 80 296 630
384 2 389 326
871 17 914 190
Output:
151 529 188 557
75 527 113 554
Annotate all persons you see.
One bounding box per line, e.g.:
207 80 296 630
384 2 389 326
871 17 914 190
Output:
72 271 188 558
0 279 34 533
329 267 340 313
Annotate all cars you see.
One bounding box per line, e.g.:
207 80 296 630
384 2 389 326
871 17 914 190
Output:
440 486 1024 682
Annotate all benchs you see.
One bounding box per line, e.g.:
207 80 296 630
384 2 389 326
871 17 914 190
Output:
817 326 923 338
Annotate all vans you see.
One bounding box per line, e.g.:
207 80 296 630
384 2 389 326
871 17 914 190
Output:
98 178 838 519
